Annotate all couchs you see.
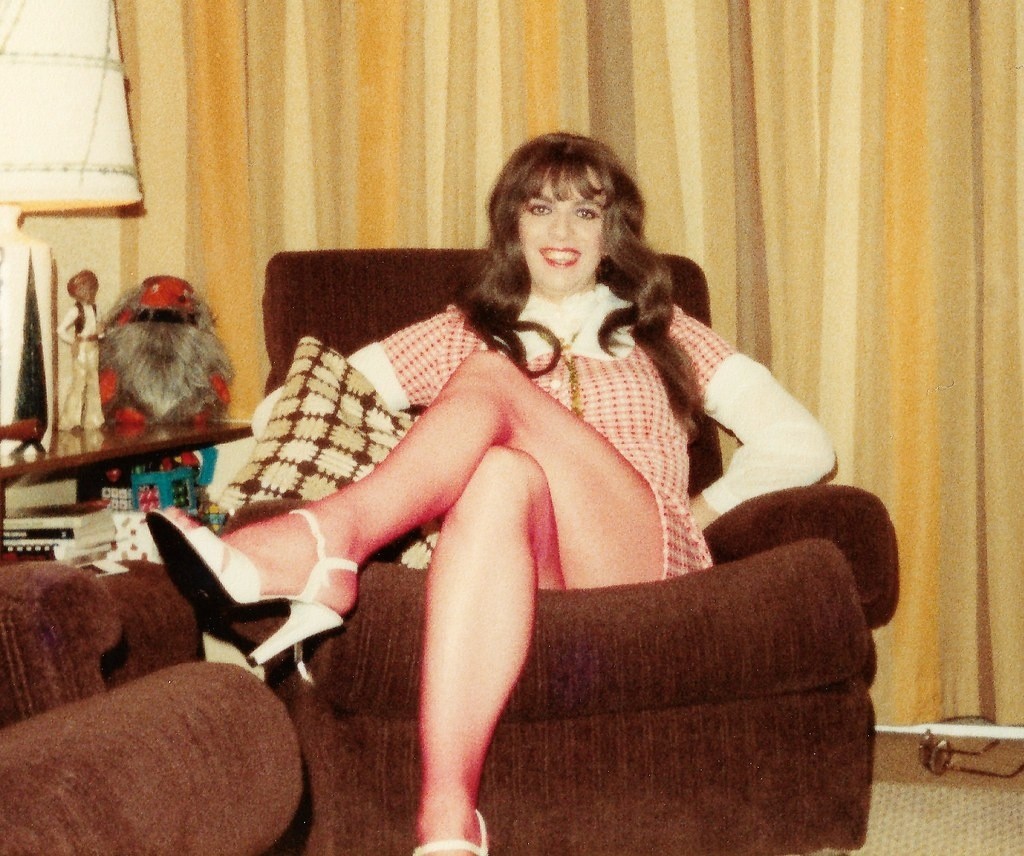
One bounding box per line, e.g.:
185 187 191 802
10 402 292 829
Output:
0 245 900 856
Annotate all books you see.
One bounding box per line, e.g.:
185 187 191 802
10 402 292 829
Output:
3 500 118 569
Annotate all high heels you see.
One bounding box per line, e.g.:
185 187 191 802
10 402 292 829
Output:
413 807 489 856
147 500 357 668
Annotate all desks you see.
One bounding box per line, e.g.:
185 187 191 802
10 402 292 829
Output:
0 418 253 553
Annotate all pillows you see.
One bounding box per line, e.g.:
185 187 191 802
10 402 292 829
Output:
223 338 440 566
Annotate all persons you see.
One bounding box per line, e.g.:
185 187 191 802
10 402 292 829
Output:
57 269 115 432
145 133 835 856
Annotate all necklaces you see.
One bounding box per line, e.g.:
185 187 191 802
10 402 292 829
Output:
539 315 581 421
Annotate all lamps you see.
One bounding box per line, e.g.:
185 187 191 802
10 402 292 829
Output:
0 0 143 438
